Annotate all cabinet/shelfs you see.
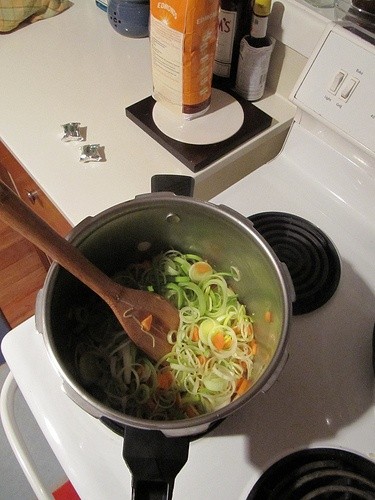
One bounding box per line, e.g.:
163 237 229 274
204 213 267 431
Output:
0 1 296 274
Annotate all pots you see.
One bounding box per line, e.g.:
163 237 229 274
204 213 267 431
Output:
35 173 297 500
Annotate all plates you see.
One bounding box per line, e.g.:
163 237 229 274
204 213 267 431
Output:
247 212 341 316
246 447 375 500
304 0 335 8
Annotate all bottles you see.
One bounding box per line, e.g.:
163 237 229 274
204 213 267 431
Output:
235 0 276 102
213 0 253 84
